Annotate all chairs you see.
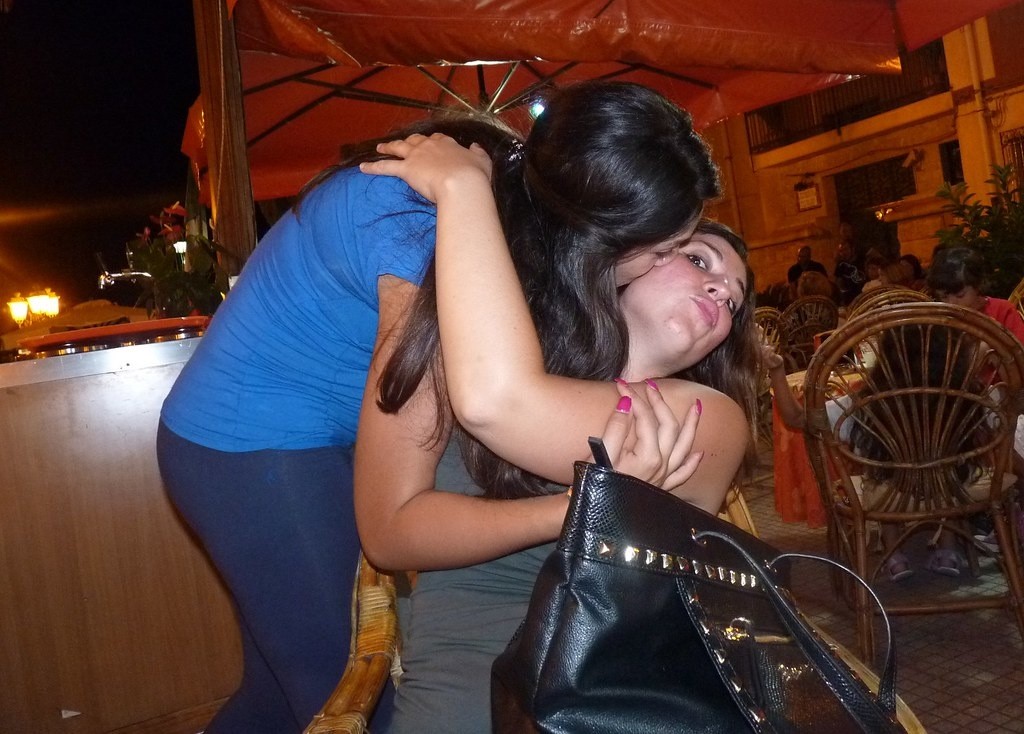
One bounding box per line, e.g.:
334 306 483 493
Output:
304 278 1023 733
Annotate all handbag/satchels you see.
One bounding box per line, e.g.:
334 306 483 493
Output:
490 438 897 734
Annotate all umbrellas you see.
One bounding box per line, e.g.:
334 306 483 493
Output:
183 1 1013 200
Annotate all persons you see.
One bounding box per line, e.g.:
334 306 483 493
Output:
835 241 867 293
155 78 759 734
759 243 1024 580
788 247 827 301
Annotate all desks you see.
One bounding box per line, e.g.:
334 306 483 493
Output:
775 360 1010 529
